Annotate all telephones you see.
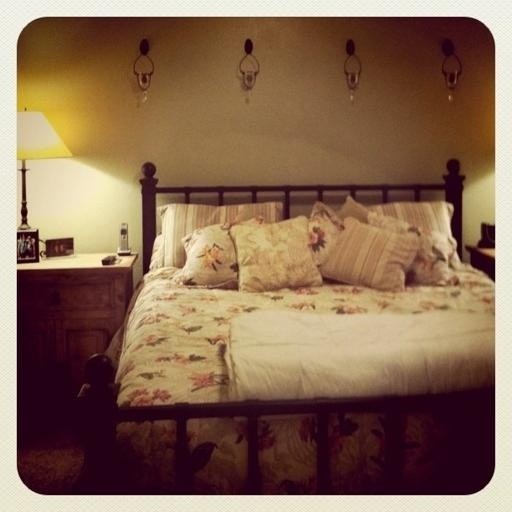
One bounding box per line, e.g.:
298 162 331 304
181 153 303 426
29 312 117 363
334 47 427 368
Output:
116 223 132 256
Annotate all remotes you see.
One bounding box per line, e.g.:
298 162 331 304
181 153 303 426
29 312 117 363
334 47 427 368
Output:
102 255 115 263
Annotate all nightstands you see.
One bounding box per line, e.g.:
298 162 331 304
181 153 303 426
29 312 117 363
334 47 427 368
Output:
17 249 140 404
463 215 496 286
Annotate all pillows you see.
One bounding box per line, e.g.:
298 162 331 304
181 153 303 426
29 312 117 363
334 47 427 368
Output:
340 195 373 225
149 200 285 271
229 214 325 292
173 217 263 290
364 199 455 243
365 211 458 288
318 216 420 291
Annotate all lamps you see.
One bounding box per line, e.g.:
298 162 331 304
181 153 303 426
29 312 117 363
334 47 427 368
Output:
18 104 74 262
77 157 496 492
